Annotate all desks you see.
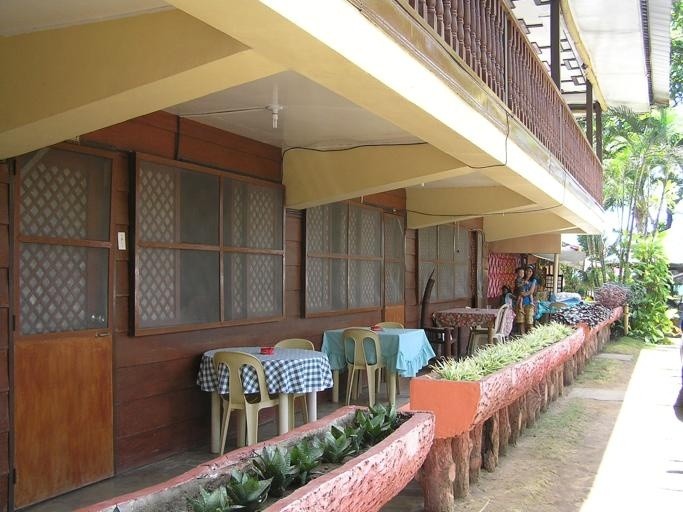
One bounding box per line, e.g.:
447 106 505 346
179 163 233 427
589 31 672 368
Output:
194 345 335 453
321 328 437 404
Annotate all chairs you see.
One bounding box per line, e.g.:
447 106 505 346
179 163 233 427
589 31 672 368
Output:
270 337 315 429
211 350 283 458
341 327 384 408
425 299 513 356
370 322 405 394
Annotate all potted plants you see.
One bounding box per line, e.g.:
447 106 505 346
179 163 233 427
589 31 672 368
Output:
70 403 436 512
568 282 630 340
406 318 585 439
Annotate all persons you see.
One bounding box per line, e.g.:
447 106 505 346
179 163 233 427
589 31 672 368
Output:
516 264 536 335
515 268 535 315
499 285 517 307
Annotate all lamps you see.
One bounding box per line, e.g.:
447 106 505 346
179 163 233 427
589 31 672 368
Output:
264 104 286 129
